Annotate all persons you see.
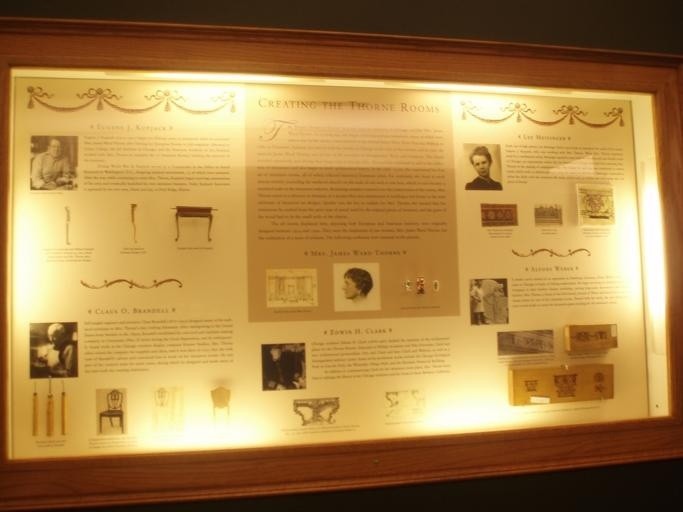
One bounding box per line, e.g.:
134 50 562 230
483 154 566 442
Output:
342 268 373 305
31 137 72 191
470 279 490 325
464 146 502 191
45 324 74 378
